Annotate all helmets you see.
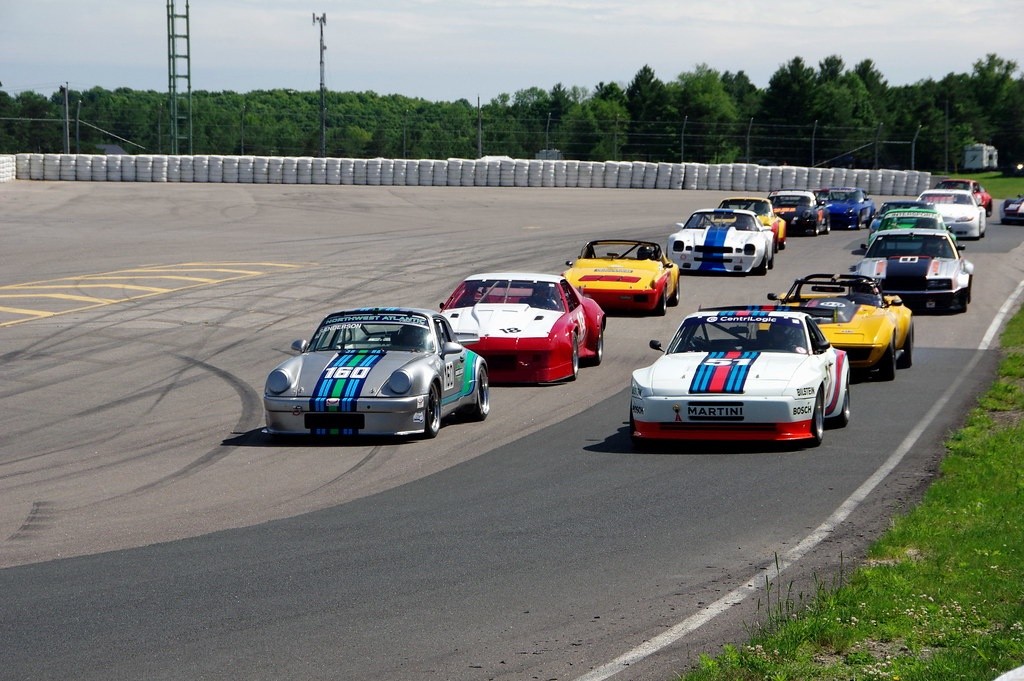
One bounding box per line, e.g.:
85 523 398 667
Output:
398 325 424 347
854 278 879 296
768 322 793 343
532 282 552 298
637 246 653 260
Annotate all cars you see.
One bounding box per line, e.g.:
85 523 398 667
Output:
812 186 875 230
867 200 935 230
916 189 985 238
869 209 956 249
998 196 1024 224
935 177 992 218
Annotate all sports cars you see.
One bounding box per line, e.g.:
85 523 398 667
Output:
852 230 974 315
766 190 830 237
628 305 851 447
562 239 681 318
668 208 774 275
261 306 490 439
718 196 787 253
434 273 607 384
767 271 915 382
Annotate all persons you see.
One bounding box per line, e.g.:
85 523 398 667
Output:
963 181 971 190
753 203 766 215
762 318 797 352
916 218 928 228
846 278 882 307
798 196 810 207
391 325 425 348
727 214 751 231
519 282 560 310
846 193 858 203
637 246 653 260
920 236 945 257
955 193 970 204
